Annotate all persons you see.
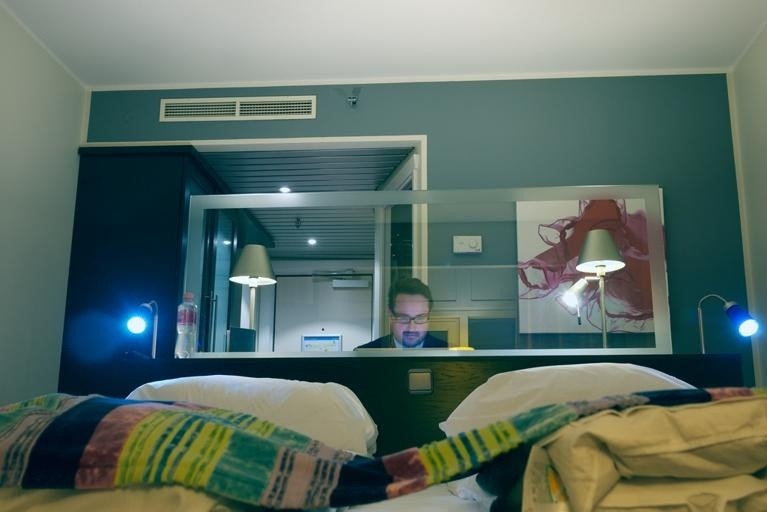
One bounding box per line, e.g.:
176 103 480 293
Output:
353 278 456 351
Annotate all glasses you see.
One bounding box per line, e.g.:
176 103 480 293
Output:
387 306 432 325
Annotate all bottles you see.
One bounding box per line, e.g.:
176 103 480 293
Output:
173 293 198 360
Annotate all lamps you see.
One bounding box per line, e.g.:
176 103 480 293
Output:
576 225 628 347
129 299 161 361
698 293 761 355
229 244 278 330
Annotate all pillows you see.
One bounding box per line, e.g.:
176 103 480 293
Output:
438 363 696 435
123 374 379 461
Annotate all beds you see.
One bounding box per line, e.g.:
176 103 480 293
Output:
1 354 765 511
